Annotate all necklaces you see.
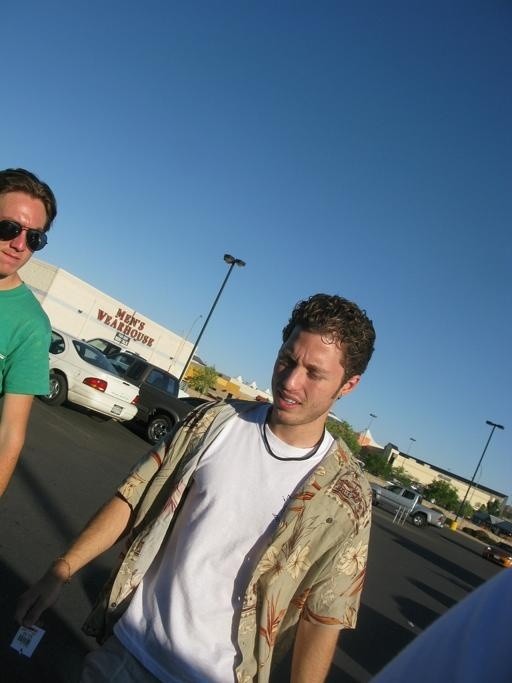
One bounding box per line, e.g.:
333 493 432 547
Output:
262 403 325 462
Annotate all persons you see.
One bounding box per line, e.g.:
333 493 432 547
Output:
367 564 511 683
13 293 386 682
0 166 57 500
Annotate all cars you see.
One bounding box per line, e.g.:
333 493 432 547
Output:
411 484 418 490
36 326 214 447
352 455 365 467
481 540 511 569
386 480 396 486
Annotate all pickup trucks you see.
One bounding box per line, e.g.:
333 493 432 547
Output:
368 483 444 529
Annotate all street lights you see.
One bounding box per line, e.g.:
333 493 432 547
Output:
178 252 246 382
447 419 505 533
401 435 416 469
360 411 377 442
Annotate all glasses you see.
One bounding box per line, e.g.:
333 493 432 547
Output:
0 219 48 251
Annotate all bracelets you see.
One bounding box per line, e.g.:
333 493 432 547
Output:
55 554 72 585
